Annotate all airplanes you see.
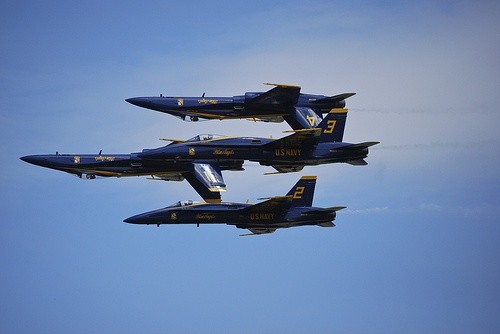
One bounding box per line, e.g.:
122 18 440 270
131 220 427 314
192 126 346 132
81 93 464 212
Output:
121 176 347 239
124 83 355 143
19 128 381 205
